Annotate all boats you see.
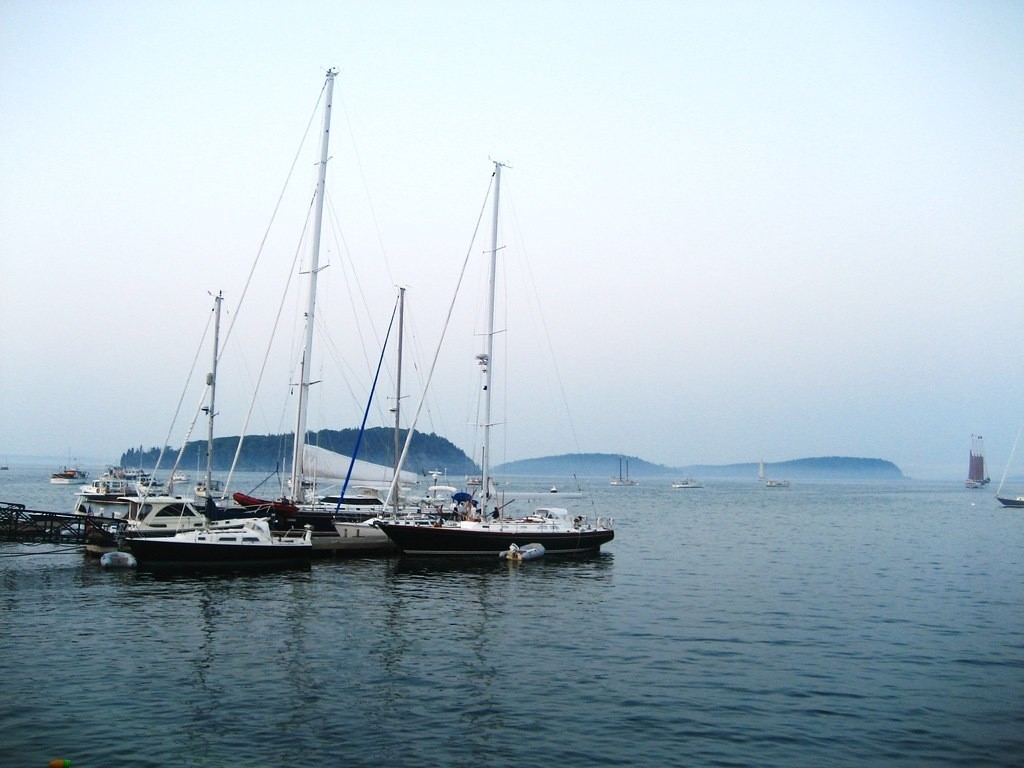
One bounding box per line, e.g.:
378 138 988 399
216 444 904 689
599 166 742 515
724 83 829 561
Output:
671 476 706 488
965 478 981 489
551 485 558 493
465 473 501 485
765 479 789 488
50 466 89 484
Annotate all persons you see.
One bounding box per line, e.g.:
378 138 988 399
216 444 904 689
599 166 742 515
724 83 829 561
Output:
281 496 286 501
492 507 499 519
451 507 458 521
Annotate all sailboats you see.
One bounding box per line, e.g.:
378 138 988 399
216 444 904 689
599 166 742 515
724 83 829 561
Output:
61 65 615 574
610 456 635 485
995 424 1024 508
